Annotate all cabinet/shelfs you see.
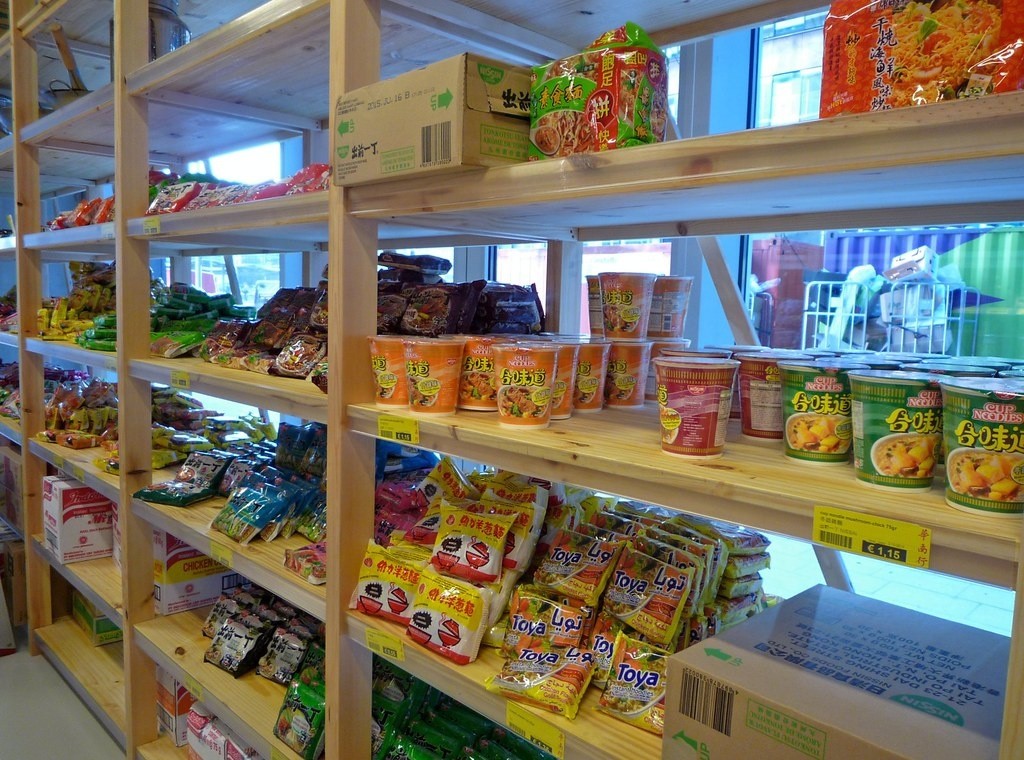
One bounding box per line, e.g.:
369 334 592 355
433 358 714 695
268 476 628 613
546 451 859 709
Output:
0 0 1023 760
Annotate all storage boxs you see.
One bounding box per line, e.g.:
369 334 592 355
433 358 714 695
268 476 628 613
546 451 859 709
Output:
0 434 75 627
151 523 254 616
69 589 123 647
331 51 537 190
112 500 122 567
42 475 113 565
660 583 1012 760
155 662 266 760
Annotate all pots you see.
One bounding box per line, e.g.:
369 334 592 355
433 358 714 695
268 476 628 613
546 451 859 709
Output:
44 80 94 111
